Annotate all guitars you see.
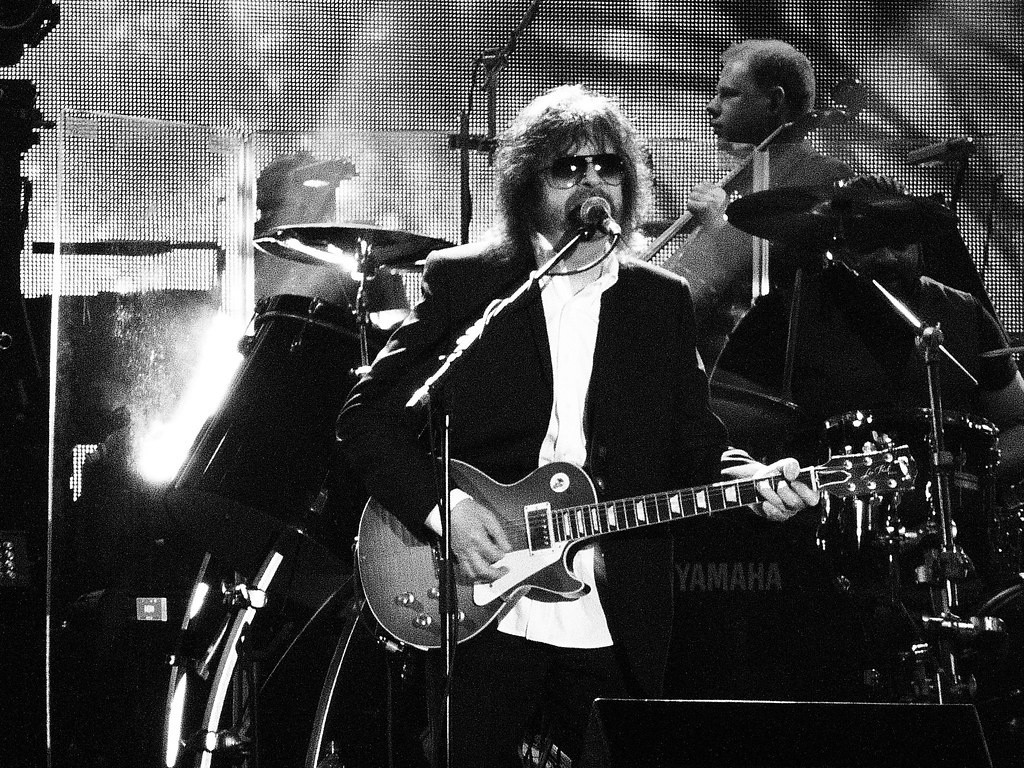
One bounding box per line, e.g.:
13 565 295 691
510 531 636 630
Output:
351 431 923 652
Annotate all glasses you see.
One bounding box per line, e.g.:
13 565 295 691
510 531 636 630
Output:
533 152 626 186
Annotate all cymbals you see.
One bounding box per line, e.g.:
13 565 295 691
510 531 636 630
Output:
724 176 884 255
253 221 463 280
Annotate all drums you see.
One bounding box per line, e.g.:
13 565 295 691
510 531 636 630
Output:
160 290 394 617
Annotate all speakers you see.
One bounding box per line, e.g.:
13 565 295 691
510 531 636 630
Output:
576 699 994 768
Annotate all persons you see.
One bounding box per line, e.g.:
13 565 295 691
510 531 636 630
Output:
698 161 1024 625
331 82 842 768
636 33 869 361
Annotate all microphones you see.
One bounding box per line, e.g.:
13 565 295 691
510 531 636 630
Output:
580 197 622 237
908 138 976 165
